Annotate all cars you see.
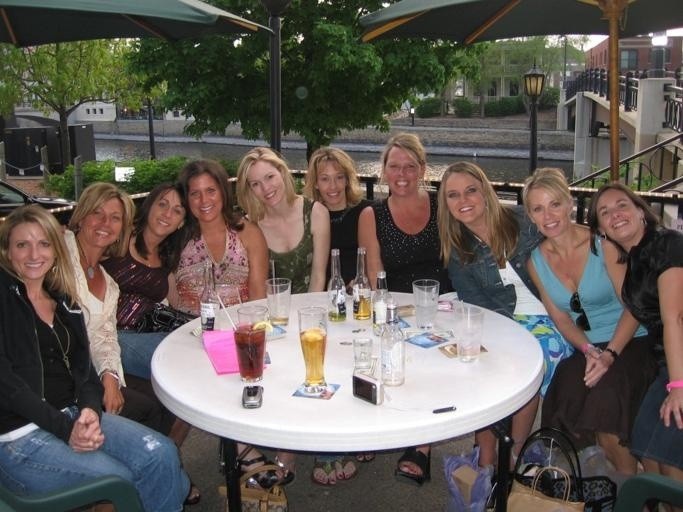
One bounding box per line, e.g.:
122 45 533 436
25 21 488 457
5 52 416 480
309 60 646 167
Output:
0 177 76 228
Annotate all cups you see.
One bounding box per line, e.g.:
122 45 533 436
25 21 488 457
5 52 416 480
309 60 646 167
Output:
265 276 291 326
297 305 328 396
412 279 440 331
236 305 268 324
351 329 374 370
233 322 265 385
452 306 485 363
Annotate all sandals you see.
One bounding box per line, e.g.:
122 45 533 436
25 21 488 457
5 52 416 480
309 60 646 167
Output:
395 448 431 488
235 447 297 488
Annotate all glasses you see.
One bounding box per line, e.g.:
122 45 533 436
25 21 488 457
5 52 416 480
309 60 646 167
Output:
569 292 590 333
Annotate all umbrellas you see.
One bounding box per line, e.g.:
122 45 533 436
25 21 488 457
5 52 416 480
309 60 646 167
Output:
356 1 682 180
1 0 267 49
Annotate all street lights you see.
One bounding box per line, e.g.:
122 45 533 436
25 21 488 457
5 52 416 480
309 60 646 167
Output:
523 55 545 174
409 105 416 126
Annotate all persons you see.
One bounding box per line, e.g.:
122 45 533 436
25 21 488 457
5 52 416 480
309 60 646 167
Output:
521 167 649 512
356 132 456 488
60 183 295 490
236 147 333 484
173 160 281 485
0 203 192 512
299 146 385 488
586 181 683 511
437 160 548 512
62 182 202 506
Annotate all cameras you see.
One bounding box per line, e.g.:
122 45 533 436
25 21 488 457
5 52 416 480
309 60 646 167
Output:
351 372 384 405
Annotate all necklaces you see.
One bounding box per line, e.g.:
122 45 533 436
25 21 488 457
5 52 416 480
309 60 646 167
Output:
75 235 99 280
331 207 350 224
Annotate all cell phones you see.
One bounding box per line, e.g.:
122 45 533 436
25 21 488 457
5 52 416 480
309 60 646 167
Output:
438 300 455 313
242 385 264 408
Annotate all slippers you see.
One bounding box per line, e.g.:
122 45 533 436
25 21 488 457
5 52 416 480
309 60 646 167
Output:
312 460 361 482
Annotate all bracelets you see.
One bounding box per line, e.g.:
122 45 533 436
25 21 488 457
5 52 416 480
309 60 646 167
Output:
605 348 621 363
579 343 594 355
664 379 683 393
99 369 122 390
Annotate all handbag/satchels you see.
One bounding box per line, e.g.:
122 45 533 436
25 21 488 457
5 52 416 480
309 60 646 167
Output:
135 303 197 334
493 470 617 512
219 484 288 512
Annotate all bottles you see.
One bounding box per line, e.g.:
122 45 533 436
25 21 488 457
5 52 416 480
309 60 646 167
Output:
326 248 347 322
199 257 220 336
382 296 406 387
352 246 371 320
372 270 391 337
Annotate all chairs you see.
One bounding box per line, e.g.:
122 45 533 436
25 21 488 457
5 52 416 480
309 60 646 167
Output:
614 473 682 511
0 471 145 512
